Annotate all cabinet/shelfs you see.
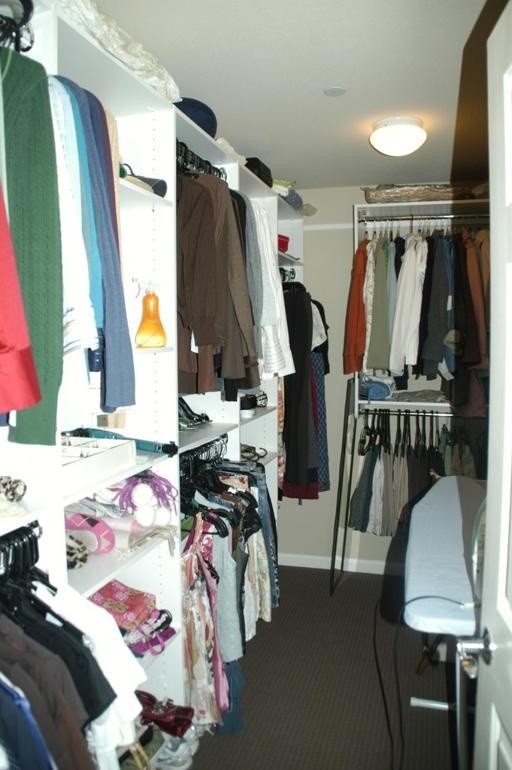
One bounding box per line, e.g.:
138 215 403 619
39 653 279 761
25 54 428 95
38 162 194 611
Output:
353 199 490 418
0 2 188 769
249 175 306 525
180 141 278 531
2 0 70 770
107 104 243 712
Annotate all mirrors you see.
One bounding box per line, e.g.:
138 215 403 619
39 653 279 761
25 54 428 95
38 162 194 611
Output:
329 378 357 595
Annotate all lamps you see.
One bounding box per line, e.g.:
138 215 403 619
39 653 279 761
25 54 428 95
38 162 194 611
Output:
370 115 428 157
134 94 167 349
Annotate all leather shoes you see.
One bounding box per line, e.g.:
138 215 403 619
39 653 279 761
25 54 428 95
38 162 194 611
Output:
178 396 212 430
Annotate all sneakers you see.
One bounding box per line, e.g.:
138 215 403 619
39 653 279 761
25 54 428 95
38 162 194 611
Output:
153 727 200 770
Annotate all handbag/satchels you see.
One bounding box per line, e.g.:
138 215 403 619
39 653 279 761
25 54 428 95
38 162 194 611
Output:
89 578 175 655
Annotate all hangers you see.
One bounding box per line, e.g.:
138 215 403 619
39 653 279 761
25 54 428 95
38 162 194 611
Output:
180 433 268 538
361 214 489 242
279 266 289 294
176 137 228 182
0 523 94 650
359 409 488 461
